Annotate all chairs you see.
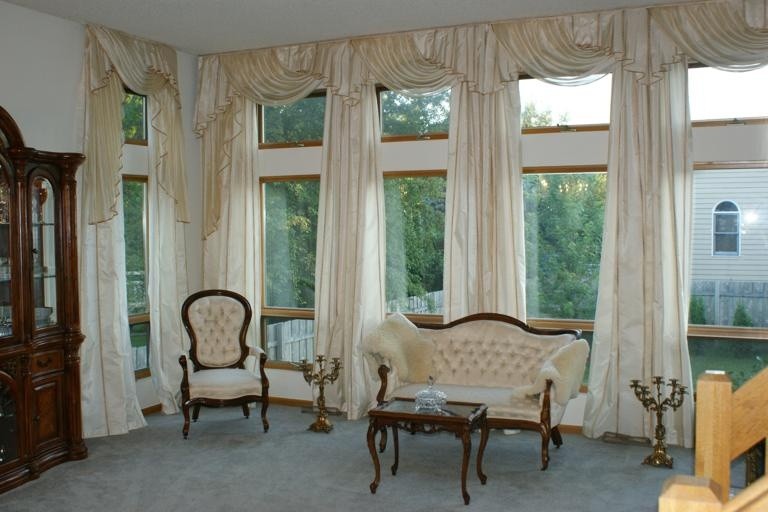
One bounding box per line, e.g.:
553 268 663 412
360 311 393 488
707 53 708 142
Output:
178 288 270 440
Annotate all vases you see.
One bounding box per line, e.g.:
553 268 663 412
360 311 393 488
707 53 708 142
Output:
414 375 447 413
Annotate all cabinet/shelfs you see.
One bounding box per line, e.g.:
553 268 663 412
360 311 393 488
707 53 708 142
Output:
0 108 88 492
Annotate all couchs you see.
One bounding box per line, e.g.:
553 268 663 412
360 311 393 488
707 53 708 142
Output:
370 312 583 471
